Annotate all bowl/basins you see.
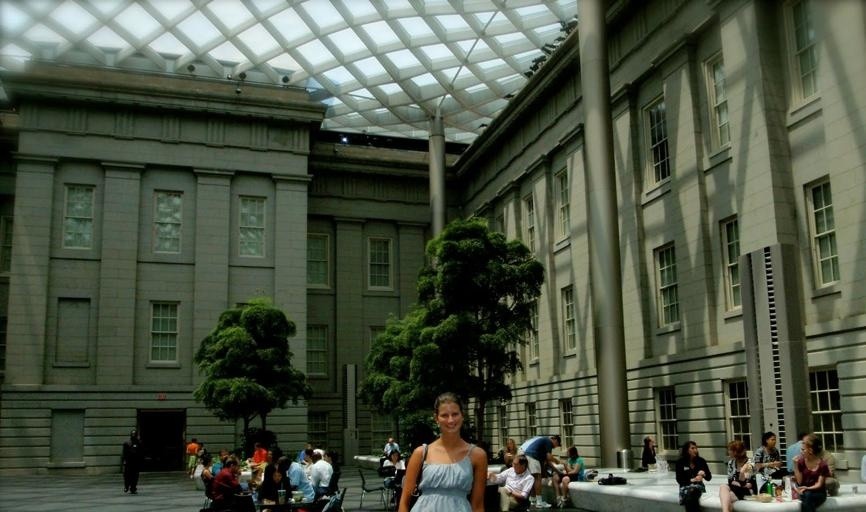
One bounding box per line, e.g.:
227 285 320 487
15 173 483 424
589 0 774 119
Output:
292 491 304 501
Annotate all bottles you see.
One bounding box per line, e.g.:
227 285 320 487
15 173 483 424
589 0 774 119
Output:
767 482 776 496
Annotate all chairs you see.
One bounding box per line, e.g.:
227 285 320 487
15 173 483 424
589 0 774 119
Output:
200 464 421 512
508 484 533 512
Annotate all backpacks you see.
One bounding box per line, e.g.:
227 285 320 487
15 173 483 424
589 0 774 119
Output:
598 473 627 485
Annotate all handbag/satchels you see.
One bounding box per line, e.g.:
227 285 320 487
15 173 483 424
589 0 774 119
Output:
395 476 419 510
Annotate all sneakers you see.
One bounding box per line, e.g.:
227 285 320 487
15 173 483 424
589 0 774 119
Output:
530 496 570 508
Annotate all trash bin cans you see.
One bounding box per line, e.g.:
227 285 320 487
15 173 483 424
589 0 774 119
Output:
617 449 634 468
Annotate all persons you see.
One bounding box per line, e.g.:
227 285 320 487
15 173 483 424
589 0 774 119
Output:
752 431 789 480
382 449 405 489
119 429 142 494
384 438 399 458
786 433 840 512
674 441 711 512
718 440 757 512
396 392 488 512
486 435 584 512
186 437 343 512
641 436 656 467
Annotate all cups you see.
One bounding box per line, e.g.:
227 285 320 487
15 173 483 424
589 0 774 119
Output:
277 489 286 503
